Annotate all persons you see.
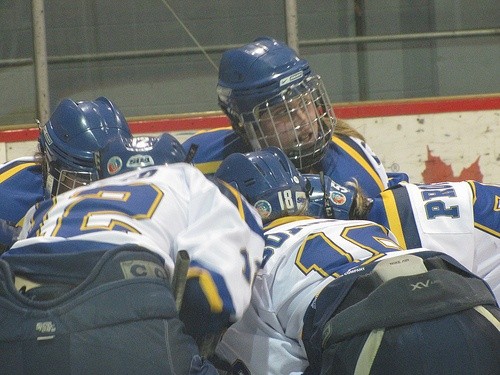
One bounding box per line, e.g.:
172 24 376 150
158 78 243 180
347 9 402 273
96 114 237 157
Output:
188 37 389 196
303 174 500 308
209 144 500 375
0 132 265 375
0 96 132 243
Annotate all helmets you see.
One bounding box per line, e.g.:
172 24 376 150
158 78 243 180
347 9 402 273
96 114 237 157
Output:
298 172 359 220
97 131 194 182
216 35 337 172
206 145 315 227
37 96 133 202
